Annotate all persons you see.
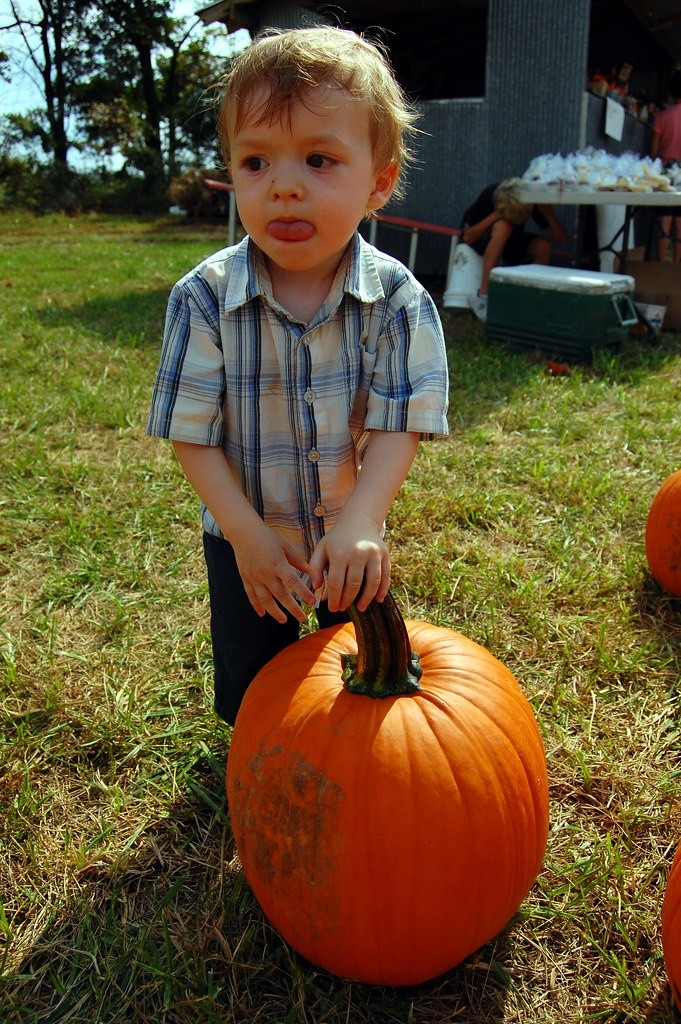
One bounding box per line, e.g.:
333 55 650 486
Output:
458 177 569 326
145 29 450 886
651 99 681 264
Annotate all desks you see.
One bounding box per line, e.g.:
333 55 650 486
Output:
518 190 681 346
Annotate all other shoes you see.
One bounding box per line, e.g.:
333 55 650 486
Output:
468 292 488 323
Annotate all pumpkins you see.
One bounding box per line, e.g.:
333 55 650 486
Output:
661 838 681 1013
644 469 681 599
225 568 548 985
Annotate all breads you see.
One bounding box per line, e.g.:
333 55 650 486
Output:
614 174 670 193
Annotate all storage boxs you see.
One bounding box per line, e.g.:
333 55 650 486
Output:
613 246 681 331
484 263 639 367
